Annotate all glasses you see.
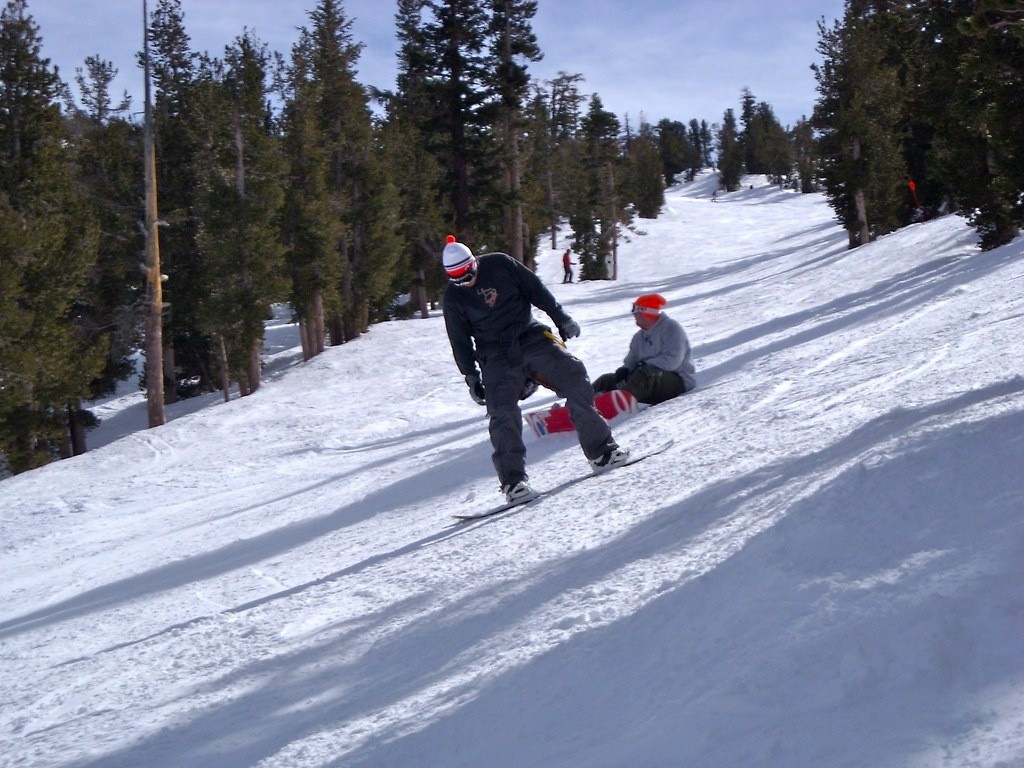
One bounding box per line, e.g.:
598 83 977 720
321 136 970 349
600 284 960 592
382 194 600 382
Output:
449 266 476 286
632 303 662 316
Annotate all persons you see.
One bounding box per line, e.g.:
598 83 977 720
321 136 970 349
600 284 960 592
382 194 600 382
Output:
591 294 696 405
443 234 627 501
711 190 717 202
562 249 577 283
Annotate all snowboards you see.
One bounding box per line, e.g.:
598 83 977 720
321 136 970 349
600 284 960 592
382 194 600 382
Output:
453 437 676 518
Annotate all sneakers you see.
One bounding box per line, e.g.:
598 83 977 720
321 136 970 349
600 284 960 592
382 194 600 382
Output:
589 447 631 471
499 473 540 504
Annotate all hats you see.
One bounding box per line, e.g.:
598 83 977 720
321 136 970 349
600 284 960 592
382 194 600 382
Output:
633 294 667 320
441 235 475 279
